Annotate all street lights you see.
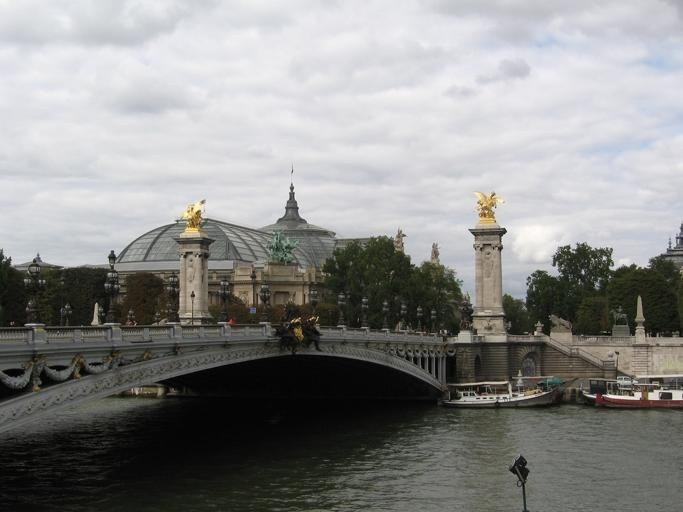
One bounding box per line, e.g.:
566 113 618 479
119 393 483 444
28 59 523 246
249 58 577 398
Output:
104 249 120 321
308 285 318 322
218 277 230 322
358 294 437 332
256 280 271 321
168 271 177 321
63 302 162 326
190 291 196 324
23 258 46 322
337 287 346 327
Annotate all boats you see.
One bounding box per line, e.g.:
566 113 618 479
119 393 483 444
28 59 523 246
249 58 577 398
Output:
441 370 578 408
582 375 683 409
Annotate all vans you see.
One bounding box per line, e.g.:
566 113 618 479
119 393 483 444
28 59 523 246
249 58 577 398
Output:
616 376 638 387
